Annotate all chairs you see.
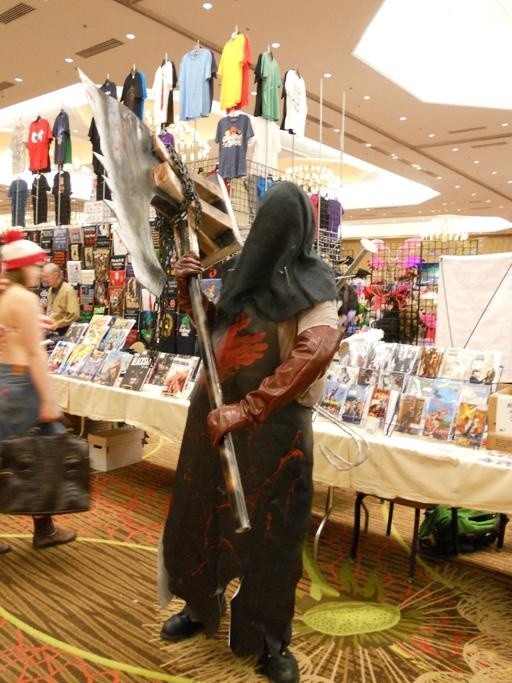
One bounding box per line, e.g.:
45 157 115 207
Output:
350 493 459 581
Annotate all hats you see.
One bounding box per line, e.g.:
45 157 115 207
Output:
0 227 47 270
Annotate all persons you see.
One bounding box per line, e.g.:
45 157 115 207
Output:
1 228 79 556
40 261 81 337
157 178 343 683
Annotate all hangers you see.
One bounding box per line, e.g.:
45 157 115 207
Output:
35 173 42 178
33 114 42 122
294 64 300 76
58 109 64 115
130 68 137 76
57 168 65 174
268 50 275 59
190 45 200 54
228 107 239 120
103 79 111 85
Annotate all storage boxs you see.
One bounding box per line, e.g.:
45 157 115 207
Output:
88 427 146 473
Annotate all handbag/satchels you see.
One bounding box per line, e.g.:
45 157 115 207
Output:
418 505 509 554
0 417 90 514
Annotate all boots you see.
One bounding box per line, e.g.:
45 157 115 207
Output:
0 544 11 554
33 516 76 546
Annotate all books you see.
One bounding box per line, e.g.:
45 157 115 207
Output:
45 314 204 399
311 334 503 454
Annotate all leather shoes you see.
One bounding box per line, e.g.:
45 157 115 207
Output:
162 591 226 640
262 647 300 682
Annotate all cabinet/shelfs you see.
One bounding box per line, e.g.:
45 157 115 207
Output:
47 373 511 560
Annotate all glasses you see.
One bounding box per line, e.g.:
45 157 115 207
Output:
32 263 46 269
